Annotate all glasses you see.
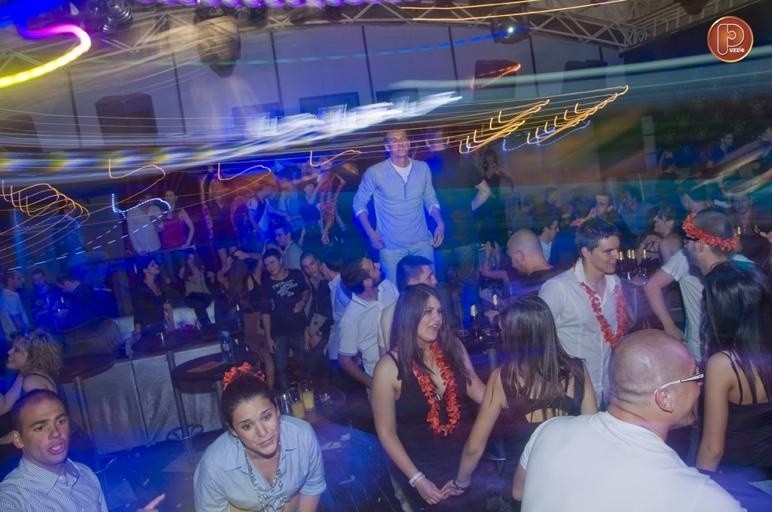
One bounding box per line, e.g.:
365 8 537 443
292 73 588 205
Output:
654 366 706 400
682 235 697 244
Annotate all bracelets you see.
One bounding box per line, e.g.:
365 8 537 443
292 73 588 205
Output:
408 471 425 488
454 479 471 490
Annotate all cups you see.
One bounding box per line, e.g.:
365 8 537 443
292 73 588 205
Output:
276 377 315 419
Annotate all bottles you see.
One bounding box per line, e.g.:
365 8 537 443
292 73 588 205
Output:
491 294 500 311
641 248 650 280
469 304 480 346
736 224 743 237
618 248 637 281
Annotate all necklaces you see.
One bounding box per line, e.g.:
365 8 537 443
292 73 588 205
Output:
243 442 289 512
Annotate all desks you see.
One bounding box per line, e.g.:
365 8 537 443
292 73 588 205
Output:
55 342 139 511
47 319 250 453
263 374 346 427
130 328 206 474
172 352 246 432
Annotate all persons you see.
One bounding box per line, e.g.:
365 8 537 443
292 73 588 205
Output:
441 293 598 512
193 363 327 512
0 389 166 512
1 330 96 467
512 329 748 512
371 282 488 511
2 118 772 413
695 259 772 477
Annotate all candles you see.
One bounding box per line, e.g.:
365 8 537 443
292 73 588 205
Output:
618 248 648 260
493 294 498 307
470 304 476 317
